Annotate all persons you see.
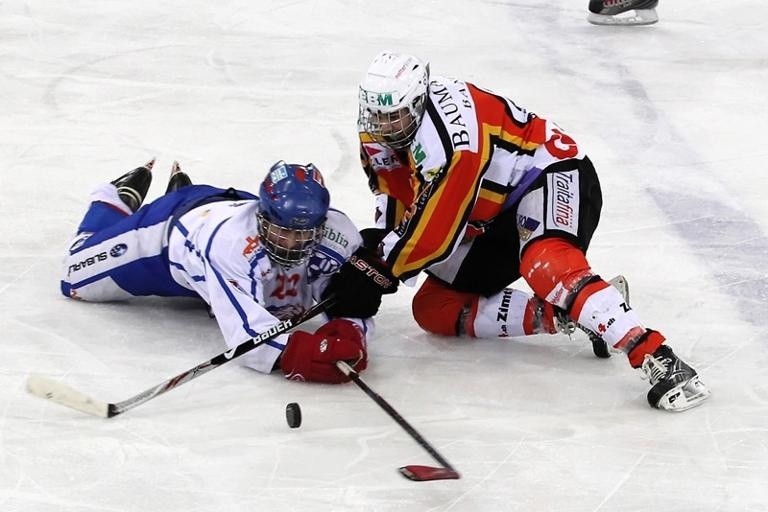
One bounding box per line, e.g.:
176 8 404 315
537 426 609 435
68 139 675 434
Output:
318 51 711 412
59 153 376 382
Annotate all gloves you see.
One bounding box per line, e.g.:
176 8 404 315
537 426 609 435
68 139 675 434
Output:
280 319 367 383
321 247 398 319
360 228 393 250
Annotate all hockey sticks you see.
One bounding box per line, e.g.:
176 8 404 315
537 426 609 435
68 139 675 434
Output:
337 361 459 482
26 295 337 419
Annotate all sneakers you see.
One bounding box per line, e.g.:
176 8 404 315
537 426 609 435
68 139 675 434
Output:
117 167 152 210
639 343 697 406
590 0 658 14
554 305 609 358
165 174 193 194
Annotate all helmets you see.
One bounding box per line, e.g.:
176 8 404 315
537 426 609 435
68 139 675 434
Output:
357 50 428 150
257 160 329 269
